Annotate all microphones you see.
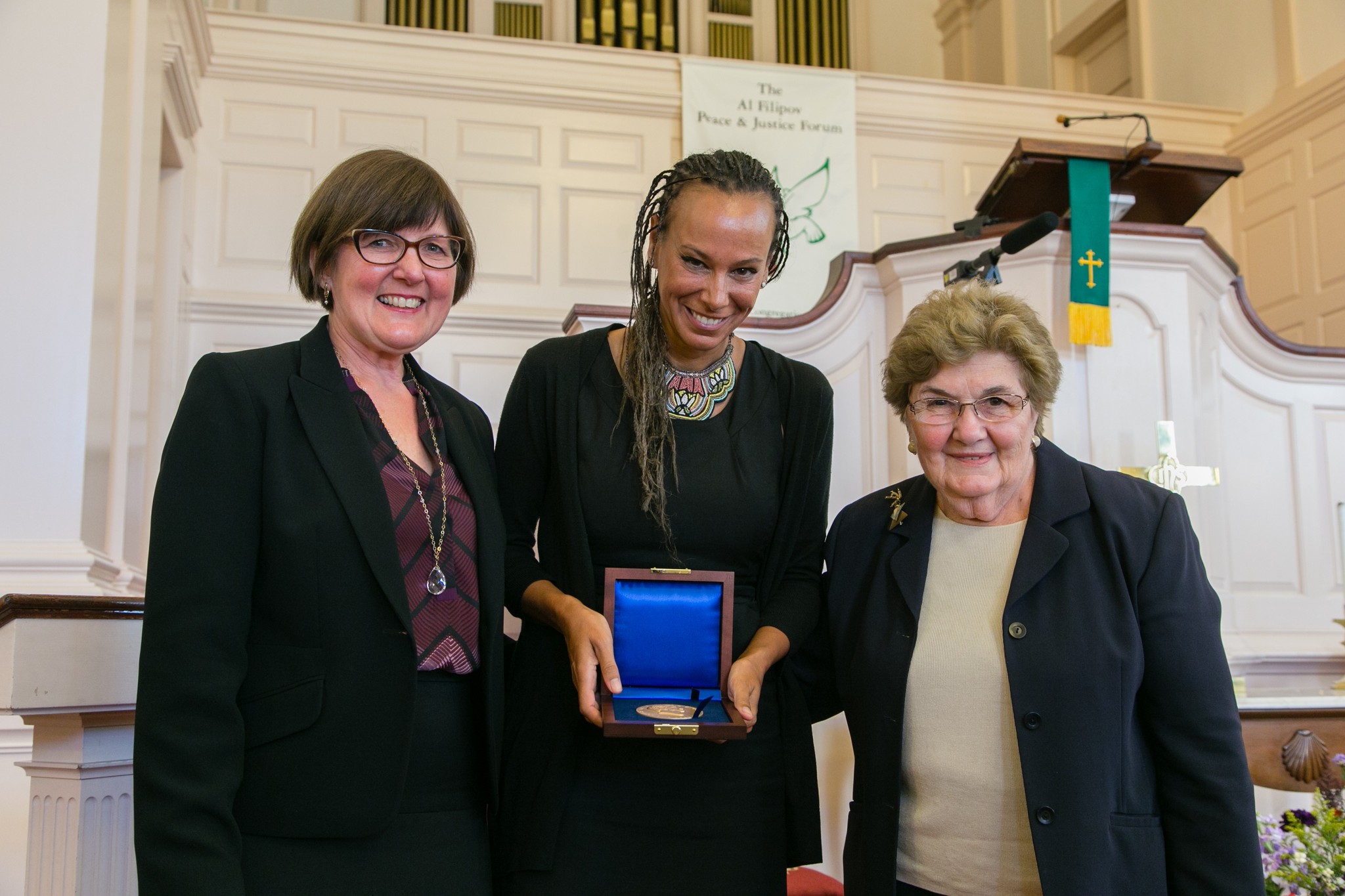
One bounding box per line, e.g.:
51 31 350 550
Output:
943 210 1060 287
1057 113 1153 142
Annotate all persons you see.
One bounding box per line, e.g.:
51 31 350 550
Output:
332 340 450 595
128 144 498 896
479 146 841 896
802 277 1270 896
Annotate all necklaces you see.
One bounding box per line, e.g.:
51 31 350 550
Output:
628 327 737 424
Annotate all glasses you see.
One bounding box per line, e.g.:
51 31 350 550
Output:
343 229 465 269
908 392 1029 425
